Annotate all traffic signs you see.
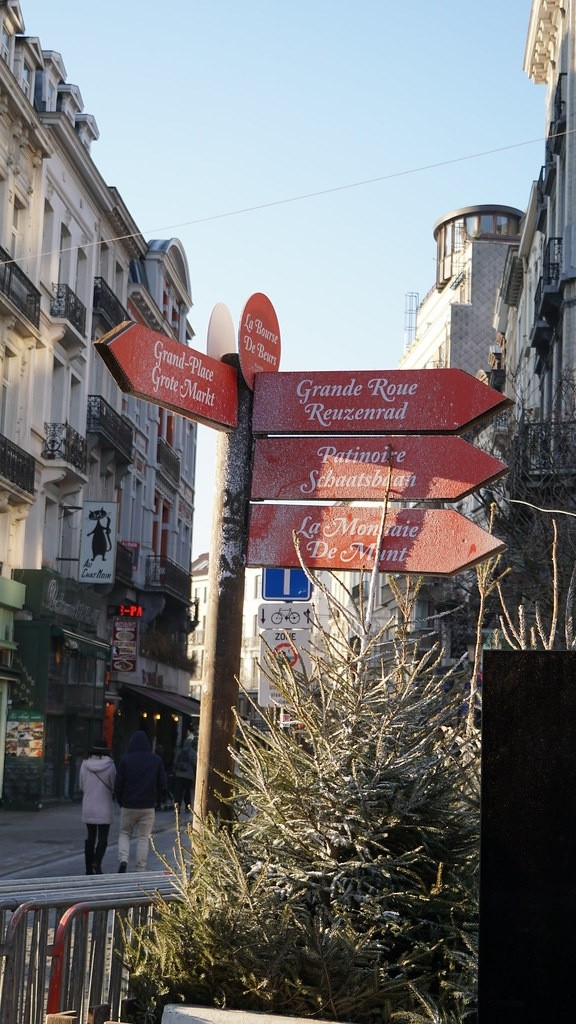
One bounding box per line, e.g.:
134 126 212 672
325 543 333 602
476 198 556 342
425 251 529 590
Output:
92 320 517 579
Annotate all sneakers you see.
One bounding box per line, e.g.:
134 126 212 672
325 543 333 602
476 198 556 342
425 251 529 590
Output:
116 860 127 873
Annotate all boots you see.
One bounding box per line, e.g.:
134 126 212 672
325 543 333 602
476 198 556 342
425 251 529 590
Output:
90 840 108 874
83 839 94 874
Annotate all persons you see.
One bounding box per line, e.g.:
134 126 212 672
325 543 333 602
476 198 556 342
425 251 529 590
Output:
78 738 117 875
173 738 196 814
114 731 168 873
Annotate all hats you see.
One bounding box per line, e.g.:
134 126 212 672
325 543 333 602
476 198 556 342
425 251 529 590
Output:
88 739 112 752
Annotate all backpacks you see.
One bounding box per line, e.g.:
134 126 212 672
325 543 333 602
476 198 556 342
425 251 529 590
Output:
177 746 191 771
